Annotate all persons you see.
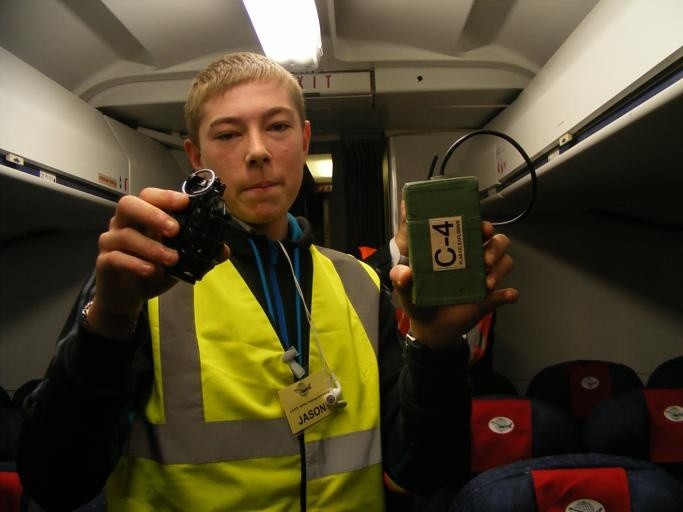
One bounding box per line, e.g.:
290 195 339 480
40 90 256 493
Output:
363 198 409 292
16 49 518 512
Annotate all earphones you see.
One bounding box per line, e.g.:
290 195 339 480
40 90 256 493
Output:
324 380 347 408
